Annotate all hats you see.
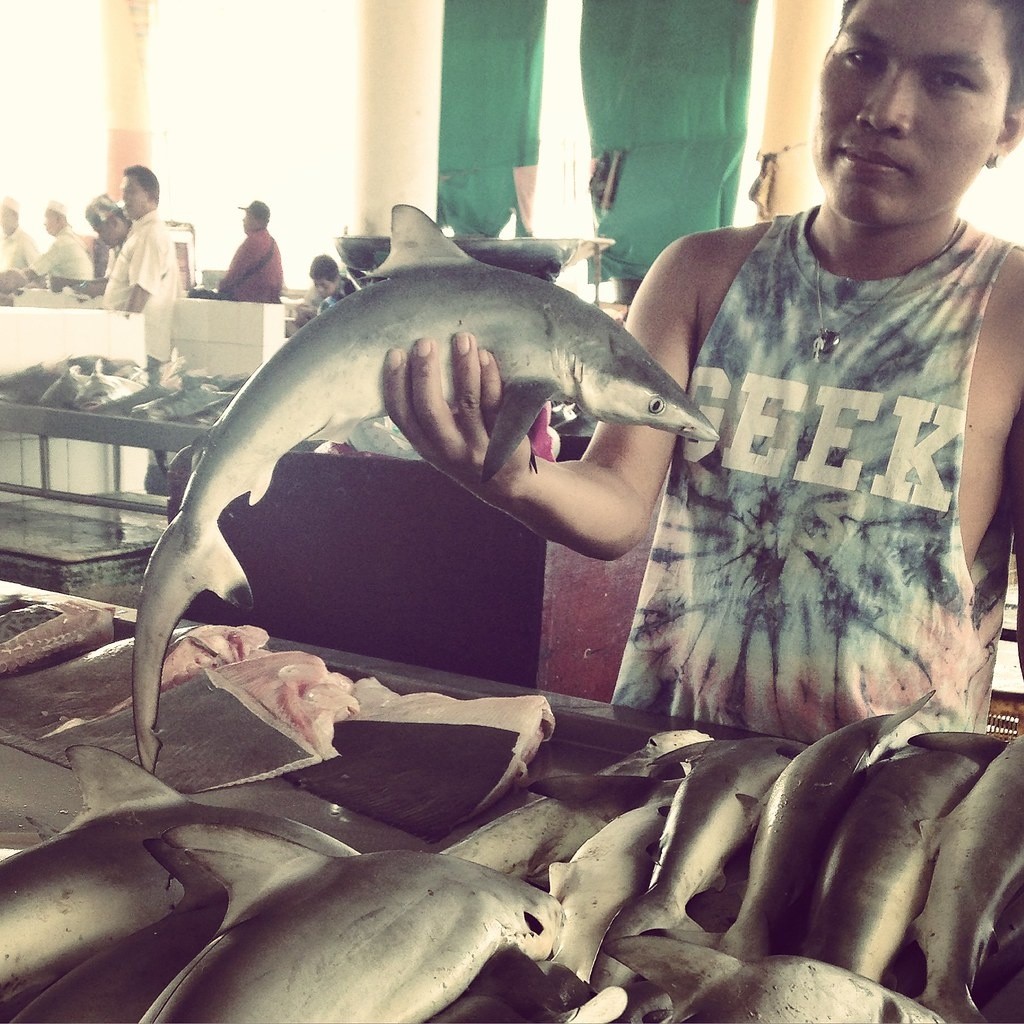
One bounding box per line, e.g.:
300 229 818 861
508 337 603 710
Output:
85 192 125 227
238 200 270 220
45 200 69 215
0 195 20 214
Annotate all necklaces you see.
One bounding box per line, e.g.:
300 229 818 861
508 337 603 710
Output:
809 215 965 353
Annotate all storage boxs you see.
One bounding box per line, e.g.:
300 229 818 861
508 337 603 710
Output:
174 298 286 374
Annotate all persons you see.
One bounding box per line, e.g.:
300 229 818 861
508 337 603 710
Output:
294 254 357 325
382 0 1024 753
0 194 135 298
101 164 179 369
217 200 283 303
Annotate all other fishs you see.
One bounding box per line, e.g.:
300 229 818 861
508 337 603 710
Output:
130 205 720 773
1 593 1024 1023
0 348 250 425
